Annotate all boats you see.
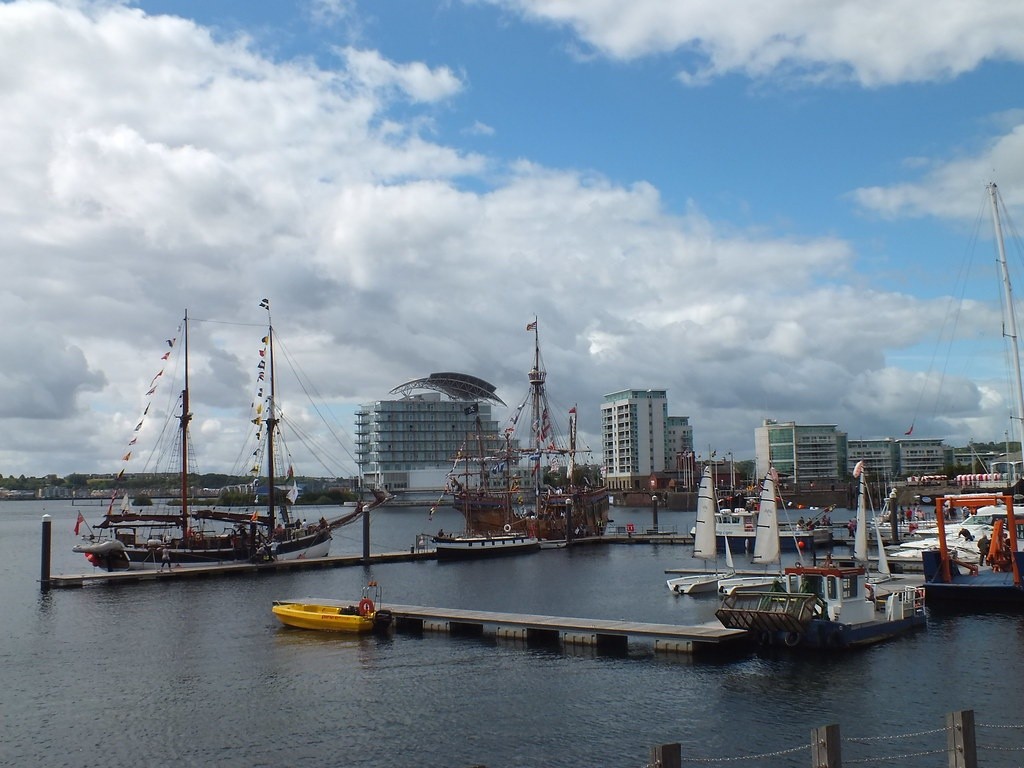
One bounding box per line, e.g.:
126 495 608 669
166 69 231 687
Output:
430 536 542 564
714 550 927 654
272 586 392 634
689 508 833 553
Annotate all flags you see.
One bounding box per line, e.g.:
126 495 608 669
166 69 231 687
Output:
677 449 716 458
286 457 298 505
569 407 575 413
776 496 836 513
429 403 559 520
251 336 268 521
74 513 83 536
106 326 181 514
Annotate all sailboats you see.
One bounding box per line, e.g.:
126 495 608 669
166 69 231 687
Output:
822 435 895 592
446 316 610 540
70 298 397 572
717 452 798 599
870 183 1024 576
666 444 737 595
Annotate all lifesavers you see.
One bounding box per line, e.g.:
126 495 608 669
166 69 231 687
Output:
194 531 202 543
360 599 375 616
915 590 923 605
783 631 803 648
504 524 512 533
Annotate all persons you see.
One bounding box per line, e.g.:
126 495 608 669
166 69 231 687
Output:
594 517 604 537
294 518 308 537
160 546 172 572
796 517 833 531
256 524 283 563
438 529 444 537
934 504 977 520
898 505 925 525
977 532 1010 566
574 524 586 538
959 528 973 541
848 516 857 539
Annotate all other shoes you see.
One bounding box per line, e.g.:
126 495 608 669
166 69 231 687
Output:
973 535 975 538
979 564 983 566
